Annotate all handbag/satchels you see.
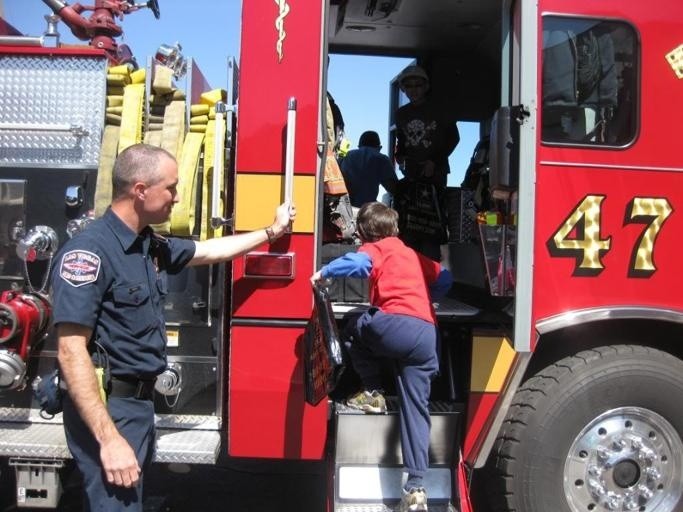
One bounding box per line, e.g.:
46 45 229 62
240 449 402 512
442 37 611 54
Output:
303 285 346 408
32 368 63 414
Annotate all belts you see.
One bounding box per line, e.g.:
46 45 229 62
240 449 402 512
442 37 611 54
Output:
101 378 154 401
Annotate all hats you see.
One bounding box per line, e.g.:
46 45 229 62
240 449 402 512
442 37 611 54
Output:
397 66 430 92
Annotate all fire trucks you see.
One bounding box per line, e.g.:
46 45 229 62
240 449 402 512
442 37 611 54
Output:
0 0 683 512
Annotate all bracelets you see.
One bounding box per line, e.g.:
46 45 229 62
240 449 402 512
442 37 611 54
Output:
265 225 274 244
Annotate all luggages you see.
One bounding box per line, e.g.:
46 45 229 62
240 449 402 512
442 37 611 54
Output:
399 180 448 245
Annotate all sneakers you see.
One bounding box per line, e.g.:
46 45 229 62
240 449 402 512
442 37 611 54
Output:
346 389 388 415
393 487 429 511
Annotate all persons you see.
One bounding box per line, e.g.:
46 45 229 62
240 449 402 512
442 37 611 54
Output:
310 202 454 510
338 129 401 217
391 64 460 248
45 136 295 510
